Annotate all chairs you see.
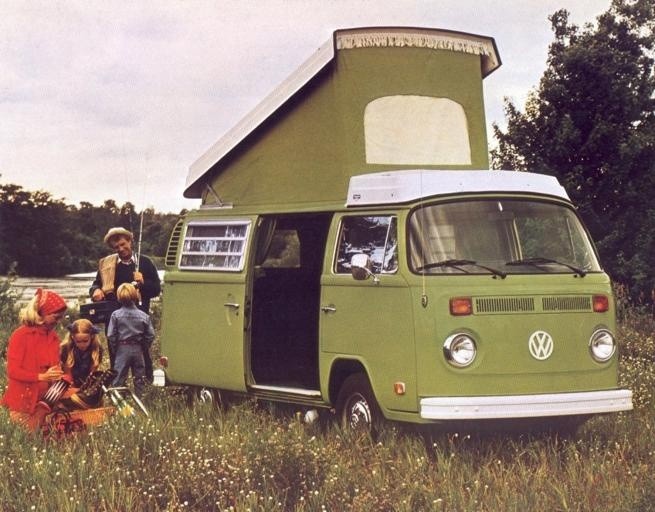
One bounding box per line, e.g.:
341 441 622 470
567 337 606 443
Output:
456 216 500 265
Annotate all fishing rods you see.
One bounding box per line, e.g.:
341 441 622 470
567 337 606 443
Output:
134 142 149 305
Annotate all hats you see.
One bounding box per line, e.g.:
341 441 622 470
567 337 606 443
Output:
104 227 135 246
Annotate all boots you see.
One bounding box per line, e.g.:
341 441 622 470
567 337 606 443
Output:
57 371 104 412
72 368 119 409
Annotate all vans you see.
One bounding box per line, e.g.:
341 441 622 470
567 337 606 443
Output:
152 26 634 432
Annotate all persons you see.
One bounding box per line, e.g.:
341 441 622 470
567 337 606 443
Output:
88 224 162 398
4 288 72 433
107 282 156 390
35 319 119 412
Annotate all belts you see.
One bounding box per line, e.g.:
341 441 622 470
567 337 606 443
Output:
118 340 141 345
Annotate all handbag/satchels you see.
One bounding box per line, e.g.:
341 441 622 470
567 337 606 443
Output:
79 302 111 323
41 419 85 440
